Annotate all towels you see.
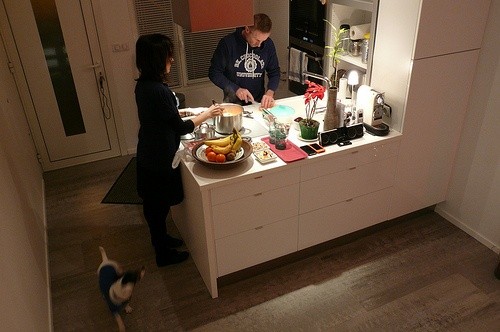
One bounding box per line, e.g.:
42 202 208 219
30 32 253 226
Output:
288 46 308 85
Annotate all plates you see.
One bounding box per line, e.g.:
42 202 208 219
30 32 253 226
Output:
254 149 277 164
195 143 245 163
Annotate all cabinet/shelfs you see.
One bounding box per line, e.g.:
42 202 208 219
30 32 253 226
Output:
210 167 301 279
321 0 380 100
298 141 397 254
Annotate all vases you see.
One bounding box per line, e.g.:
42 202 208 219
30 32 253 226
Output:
325 88 339 130
299 119 319 139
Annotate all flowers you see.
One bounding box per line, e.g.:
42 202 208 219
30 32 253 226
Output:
301 79 325 124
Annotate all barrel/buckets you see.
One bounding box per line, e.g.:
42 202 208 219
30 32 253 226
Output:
213 102 245 134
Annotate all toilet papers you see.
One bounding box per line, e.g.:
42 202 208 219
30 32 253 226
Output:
349 22 371 40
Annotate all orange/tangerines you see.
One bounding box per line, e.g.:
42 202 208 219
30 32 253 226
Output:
206 147 226 162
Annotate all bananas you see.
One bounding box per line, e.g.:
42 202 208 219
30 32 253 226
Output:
203 128 242 154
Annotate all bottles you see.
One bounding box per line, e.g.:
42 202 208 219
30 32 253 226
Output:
339 24 370 64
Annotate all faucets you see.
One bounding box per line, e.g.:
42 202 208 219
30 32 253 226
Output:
302 70 332 89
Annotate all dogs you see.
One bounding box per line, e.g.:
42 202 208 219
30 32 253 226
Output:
96 245 146 332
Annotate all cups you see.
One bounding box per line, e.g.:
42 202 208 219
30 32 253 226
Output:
268 114 291 149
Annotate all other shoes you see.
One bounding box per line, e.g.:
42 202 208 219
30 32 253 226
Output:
156 251 189 267
152 236 185 248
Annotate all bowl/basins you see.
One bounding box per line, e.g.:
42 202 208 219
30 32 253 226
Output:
192 136 253 166
194 122 215 140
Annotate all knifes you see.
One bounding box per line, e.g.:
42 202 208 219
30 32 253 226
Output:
247 96 260 105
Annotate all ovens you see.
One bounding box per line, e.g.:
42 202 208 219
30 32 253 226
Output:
287 0 328 94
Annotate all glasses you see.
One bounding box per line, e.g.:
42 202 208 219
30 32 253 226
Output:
167 58 174 64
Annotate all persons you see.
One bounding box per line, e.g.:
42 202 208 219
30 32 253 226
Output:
208 13 281 107
135 33 225 268
